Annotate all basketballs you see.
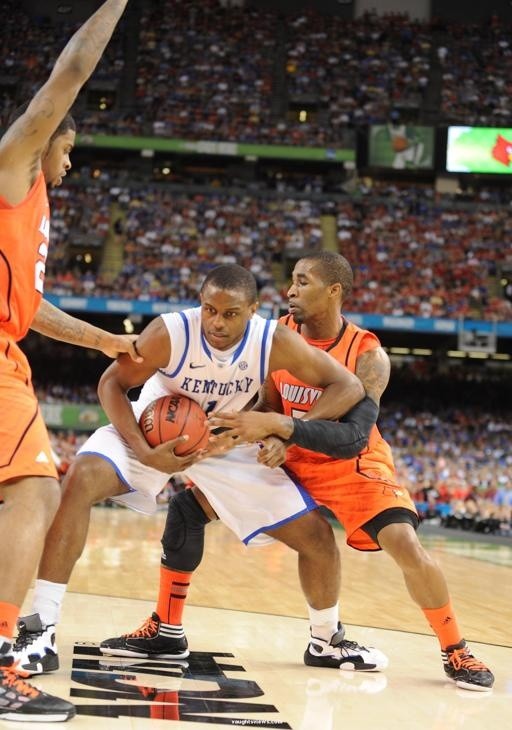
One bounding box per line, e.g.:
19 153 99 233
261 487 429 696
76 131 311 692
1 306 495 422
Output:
139 395 212 457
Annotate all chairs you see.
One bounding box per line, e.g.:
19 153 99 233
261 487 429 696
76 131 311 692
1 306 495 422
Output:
21 28 512 510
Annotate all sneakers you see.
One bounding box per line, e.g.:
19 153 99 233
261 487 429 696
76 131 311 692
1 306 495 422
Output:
304 622 385 671
0 613 76 722
441 639 494 687
100 612 190 660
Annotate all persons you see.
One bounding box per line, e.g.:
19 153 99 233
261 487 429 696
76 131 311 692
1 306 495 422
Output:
99 247 497 687
1 2 511 532
6 261 388 676
2 0 139 716
